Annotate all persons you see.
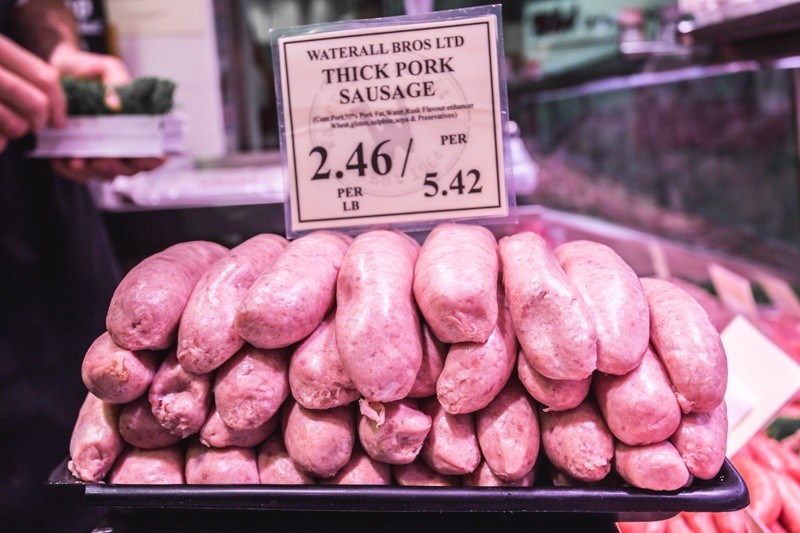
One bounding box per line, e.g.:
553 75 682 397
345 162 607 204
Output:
2 0 118 531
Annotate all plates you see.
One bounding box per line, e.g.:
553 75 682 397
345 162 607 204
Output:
48 447 751 525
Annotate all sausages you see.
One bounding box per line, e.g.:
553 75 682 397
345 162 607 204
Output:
69 222 800 533
